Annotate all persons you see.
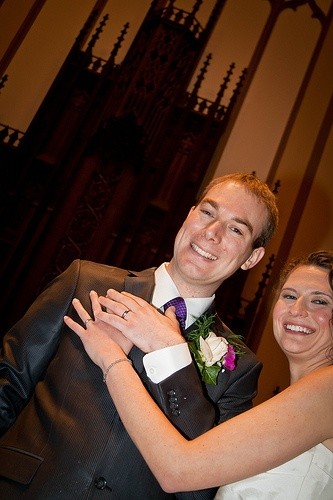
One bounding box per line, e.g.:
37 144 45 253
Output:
63 247 333 500
1 172 280 500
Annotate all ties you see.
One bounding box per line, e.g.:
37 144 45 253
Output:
164 298 187 330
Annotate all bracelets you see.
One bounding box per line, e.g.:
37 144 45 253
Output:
103 357 133 385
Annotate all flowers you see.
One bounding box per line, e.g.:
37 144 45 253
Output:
183 312 246 387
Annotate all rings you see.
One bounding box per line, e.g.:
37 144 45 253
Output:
83 318 93 326
121 309 132 318
